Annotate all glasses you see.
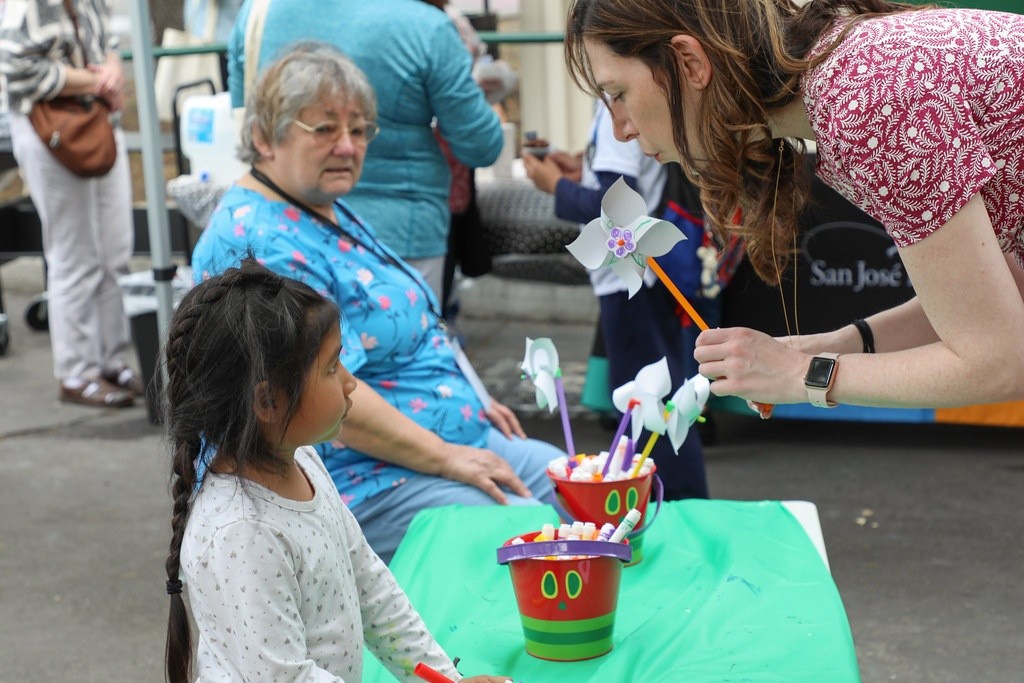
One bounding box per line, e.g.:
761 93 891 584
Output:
289 118 379 144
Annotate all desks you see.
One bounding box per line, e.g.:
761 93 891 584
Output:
363 498 861 683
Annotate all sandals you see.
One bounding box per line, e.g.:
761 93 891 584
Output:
58 365 145 407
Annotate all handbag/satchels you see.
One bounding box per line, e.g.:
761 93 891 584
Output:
26 0 117 178
230 1 270 177
155 1 228 122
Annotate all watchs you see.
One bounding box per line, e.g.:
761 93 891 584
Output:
803 352 843 409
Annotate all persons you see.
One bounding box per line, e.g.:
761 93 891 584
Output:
413 0 512 323
186 43 580 567
0 1 147 412
563 2 1024 419
159 252 515 683
519 90 710 503
224 1 504 309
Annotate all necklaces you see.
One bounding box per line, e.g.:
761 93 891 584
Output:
772 136 803 352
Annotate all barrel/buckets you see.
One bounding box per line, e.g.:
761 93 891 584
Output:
496 530 632 663
547 448 664 569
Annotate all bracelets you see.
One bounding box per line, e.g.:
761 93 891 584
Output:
852 319 877 355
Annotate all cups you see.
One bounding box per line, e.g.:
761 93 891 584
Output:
522 138 550 167
